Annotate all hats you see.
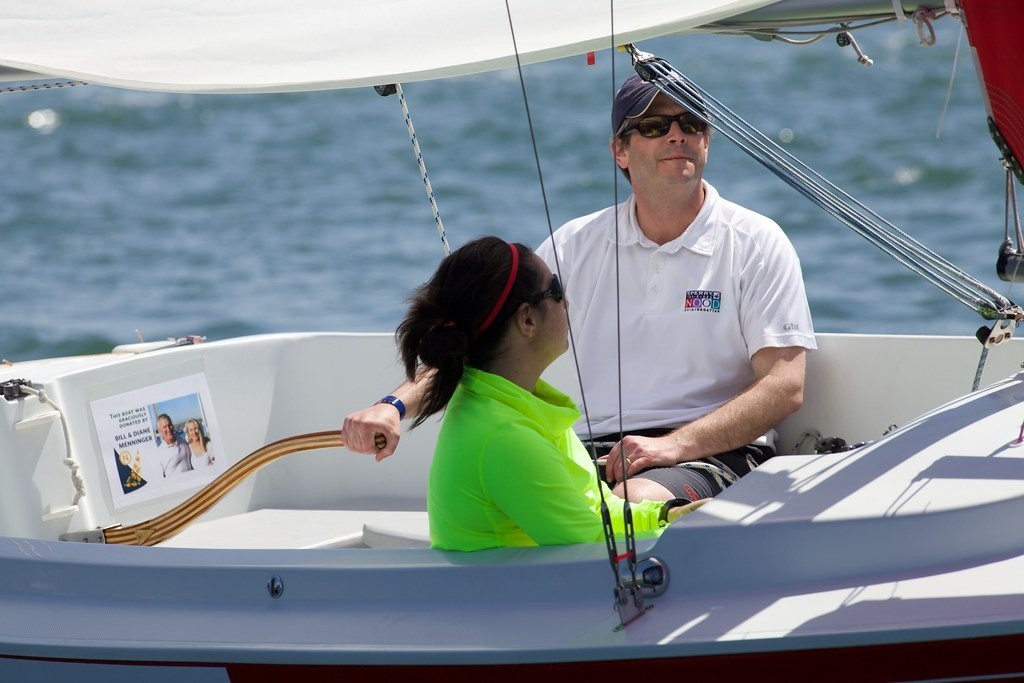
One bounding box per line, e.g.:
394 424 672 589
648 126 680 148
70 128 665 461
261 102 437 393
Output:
612 74 706 135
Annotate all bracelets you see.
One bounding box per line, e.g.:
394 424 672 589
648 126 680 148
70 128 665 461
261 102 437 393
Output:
373 396 406 421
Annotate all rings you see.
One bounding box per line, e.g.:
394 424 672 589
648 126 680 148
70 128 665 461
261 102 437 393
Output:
625 457 631 464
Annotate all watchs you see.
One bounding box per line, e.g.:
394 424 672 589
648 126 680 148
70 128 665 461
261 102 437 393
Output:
658 497 691 527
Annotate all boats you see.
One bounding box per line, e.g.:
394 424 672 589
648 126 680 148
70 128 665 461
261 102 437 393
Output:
0 0 1024 683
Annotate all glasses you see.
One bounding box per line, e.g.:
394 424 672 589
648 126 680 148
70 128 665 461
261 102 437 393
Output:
528 273 562 302
621 111 708 138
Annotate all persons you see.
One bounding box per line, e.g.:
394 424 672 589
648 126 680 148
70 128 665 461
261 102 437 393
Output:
342 74 819 504
394 236 716 553
156 413 215 478
